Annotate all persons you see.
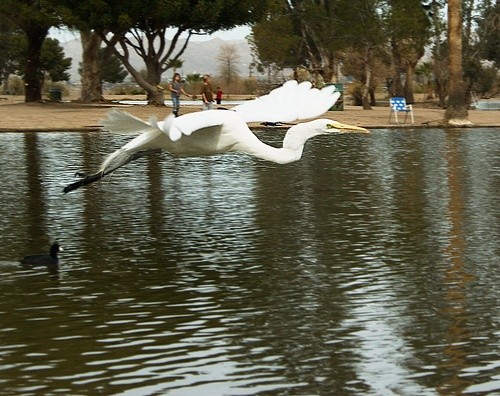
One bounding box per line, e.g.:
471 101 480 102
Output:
215 87 223 104
200 75 214 110
169 73 189 117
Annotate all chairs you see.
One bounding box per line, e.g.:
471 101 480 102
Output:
387 97 414 124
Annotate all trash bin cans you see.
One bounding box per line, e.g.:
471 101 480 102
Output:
48 87 64 102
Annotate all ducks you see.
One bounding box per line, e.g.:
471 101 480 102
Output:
20 241 64 265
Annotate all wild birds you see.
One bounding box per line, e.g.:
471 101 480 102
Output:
62 77 370 197
